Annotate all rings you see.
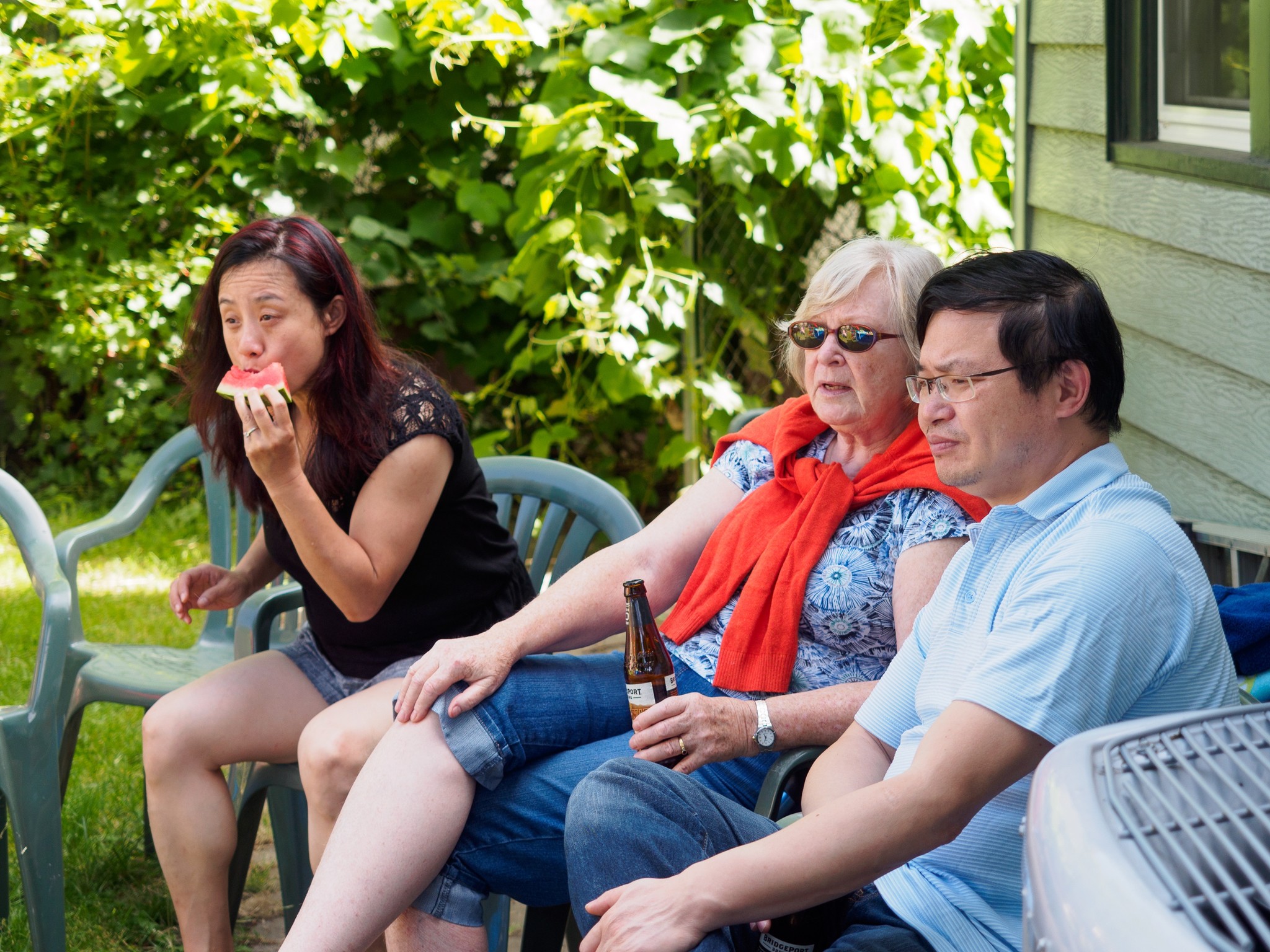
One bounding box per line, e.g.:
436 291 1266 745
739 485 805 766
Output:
678 735 687 755
243 426 258 437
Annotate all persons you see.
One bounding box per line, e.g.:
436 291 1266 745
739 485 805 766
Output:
562 250 1243 952
141 215 534 952
277 238 976 952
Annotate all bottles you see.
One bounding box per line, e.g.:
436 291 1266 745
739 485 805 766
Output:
623 579 685 769
756 907 818 952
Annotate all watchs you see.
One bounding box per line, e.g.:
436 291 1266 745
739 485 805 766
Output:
753 699 776 755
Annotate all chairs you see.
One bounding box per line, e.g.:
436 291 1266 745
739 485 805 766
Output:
55 423 304 863
228 456 646 952
0 468 73 952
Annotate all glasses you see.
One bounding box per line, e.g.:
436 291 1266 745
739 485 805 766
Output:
904 358 1047 403
787 321 904 352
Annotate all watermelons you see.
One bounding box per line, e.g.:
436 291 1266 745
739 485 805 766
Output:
216 362 292 406
